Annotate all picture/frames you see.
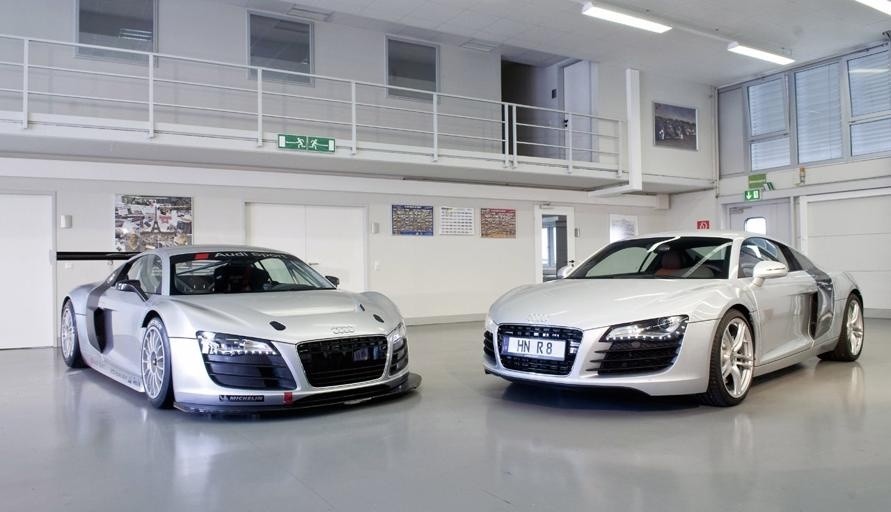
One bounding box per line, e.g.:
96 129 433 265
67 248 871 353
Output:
651 100 701 154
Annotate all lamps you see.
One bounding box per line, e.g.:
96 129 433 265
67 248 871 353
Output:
581 0 796 66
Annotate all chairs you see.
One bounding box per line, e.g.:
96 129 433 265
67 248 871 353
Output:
654 250 681 277
214 265 268 292
726 247 757 277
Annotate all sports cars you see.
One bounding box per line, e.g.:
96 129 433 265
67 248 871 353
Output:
57 241 412 416
480 231 865 409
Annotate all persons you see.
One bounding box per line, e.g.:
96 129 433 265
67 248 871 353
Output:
657 123 692 143
125 234 140 253
